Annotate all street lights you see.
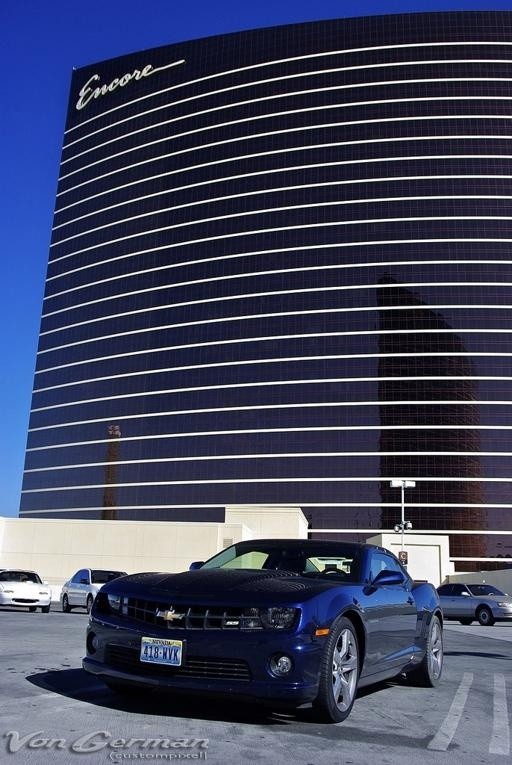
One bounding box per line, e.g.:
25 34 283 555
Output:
391 481 416 568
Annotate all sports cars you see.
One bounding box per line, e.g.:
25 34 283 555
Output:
82 537 446 725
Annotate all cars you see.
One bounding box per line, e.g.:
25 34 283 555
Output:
0 566 53 614
59 568 129 615
434 582 512 627
